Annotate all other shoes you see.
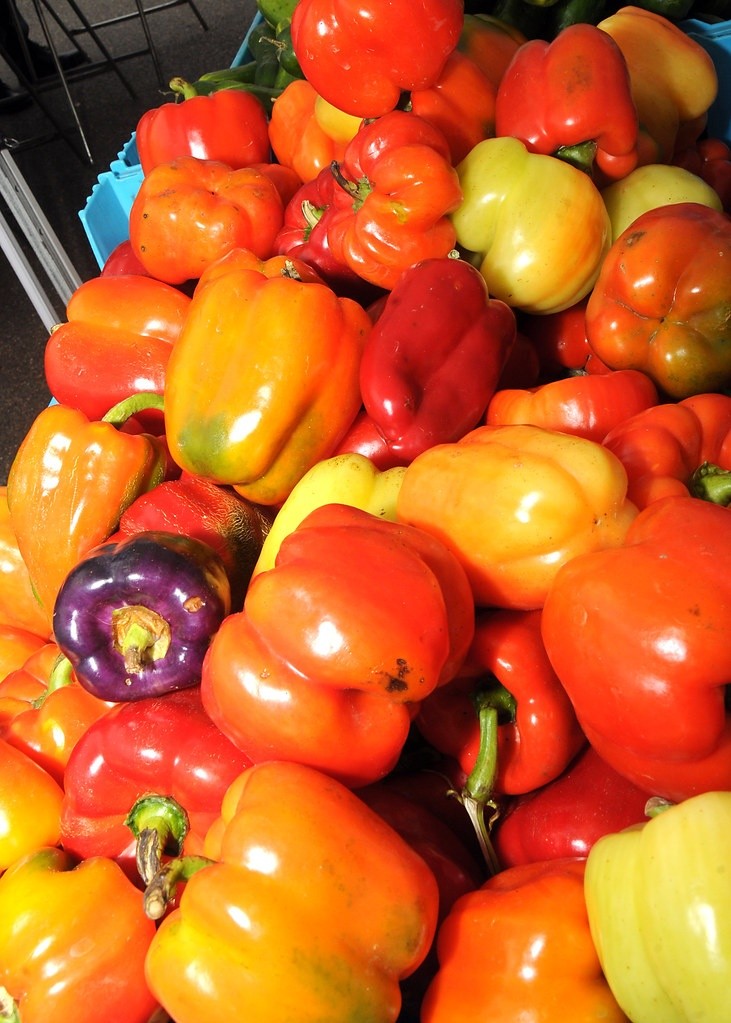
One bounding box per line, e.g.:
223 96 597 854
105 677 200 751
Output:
19 49 87 83
0 81 11 99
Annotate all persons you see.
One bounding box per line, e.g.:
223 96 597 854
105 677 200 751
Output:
0 0 88 117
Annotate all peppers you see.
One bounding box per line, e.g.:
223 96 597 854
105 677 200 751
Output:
0 0 731 1023
